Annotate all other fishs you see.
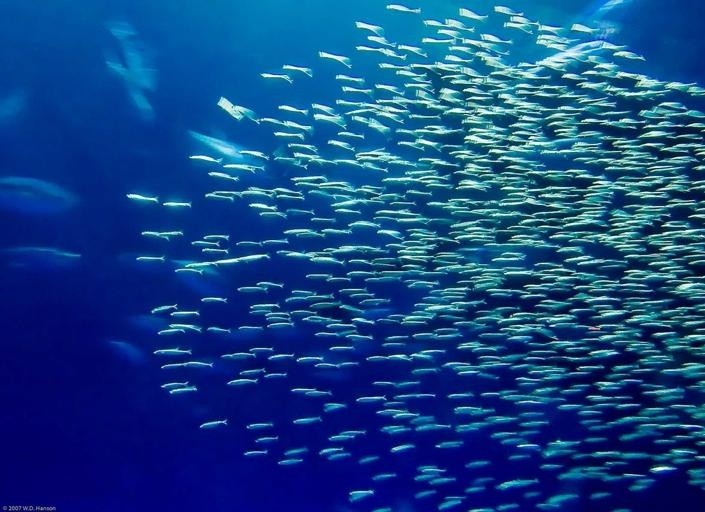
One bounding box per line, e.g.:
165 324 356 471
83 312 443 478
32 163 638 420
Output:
125 0 704 512
